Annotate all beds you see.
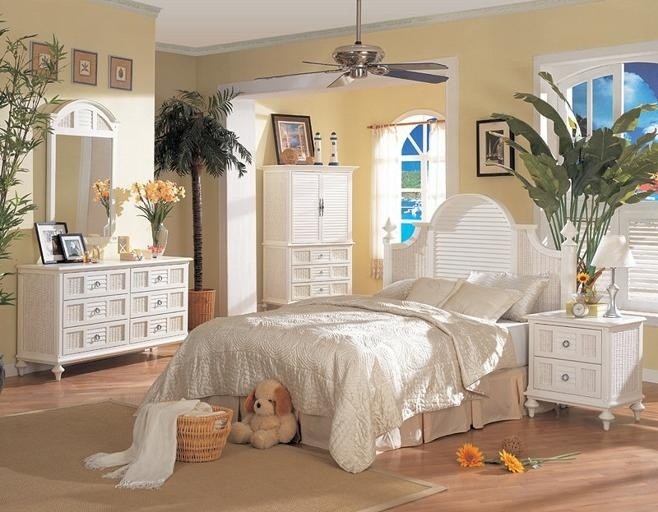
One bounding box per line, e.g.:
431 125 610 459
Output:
135 192 579 474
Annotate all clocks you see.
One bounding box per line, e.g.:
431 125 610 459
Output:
570 303 592 318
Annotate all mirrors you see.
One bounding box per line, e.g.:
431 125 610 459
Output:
45 97 120 242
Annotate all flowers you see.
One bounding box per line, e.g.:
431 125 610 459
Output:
453 439 581 474
573 271 590 300
131 178 187 246
91 177 111 216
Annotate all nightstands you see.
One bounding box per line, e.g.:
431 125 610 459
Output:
524 309 649 434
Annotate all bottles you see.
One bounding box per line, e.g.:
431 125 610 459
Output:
313 132 323 165
329 132 339 166
557 221 580 310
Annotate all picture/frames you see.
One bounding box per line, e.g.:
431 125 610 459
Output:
269 112 317 166
33 222 69 266
71 48 99 86
58 232 90 264
28 41 59 85
476 118 516 178
108 55 132 91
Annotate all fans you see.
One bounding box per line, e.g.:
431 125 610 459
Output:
253 1 451 89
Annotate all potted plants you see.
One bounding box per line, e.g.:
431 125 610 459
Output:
0 26 75 394
154 84 254 335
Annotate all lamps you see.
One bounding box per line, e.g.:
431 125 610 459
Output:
590 233 638 320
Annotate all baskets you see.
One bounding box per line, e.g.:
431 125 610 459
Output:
172 405 233 463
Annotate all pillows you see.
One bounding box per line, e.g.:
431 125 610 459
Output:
465 269 551 325
371 277 417 301
404 276 456 310
436 276 525 324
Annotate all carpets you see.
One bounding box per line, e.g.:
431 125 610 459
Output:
0 399 449 511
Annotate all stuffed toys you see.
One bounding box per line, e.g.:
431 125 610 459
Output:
226 378 297 448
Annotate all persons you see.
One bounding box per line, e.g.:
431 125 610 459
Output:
69 241 81 257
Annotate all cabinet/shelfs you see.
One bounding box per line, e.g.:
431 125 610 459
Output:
12 255 197 386
260 162 361 312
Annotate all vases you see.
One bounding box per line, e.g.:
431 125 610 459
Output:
101 218 110 237
146 224 169 255
